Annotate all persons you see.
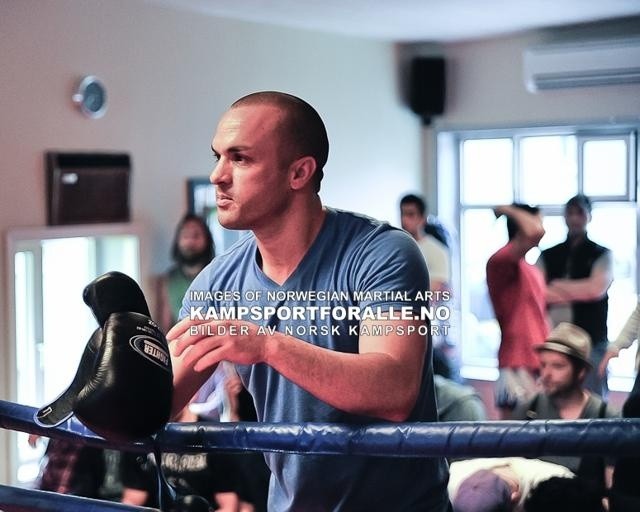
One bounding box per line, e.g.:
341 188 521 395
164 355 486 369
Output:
398 193 462 379
505 321 622 420
482 202 553 422
534 193 614 403
165 87 457 511
154 212 217 339
596 299 639 512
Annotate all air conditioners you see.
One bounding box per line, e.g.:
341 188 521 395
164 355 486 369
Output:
519 36 639 94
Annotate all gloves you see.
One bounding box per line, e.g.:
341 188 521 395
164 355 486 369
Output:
33 269 178 442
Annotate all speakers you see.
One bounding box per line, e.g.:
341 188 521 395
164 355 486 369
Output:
410 55 446 115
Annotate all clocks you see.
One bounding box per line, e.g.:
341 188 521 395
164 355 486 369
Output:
74 73 112 119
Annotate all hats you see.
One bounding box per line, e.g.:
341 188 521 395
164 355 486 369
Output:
533 320 595 372
452 469 513 511
